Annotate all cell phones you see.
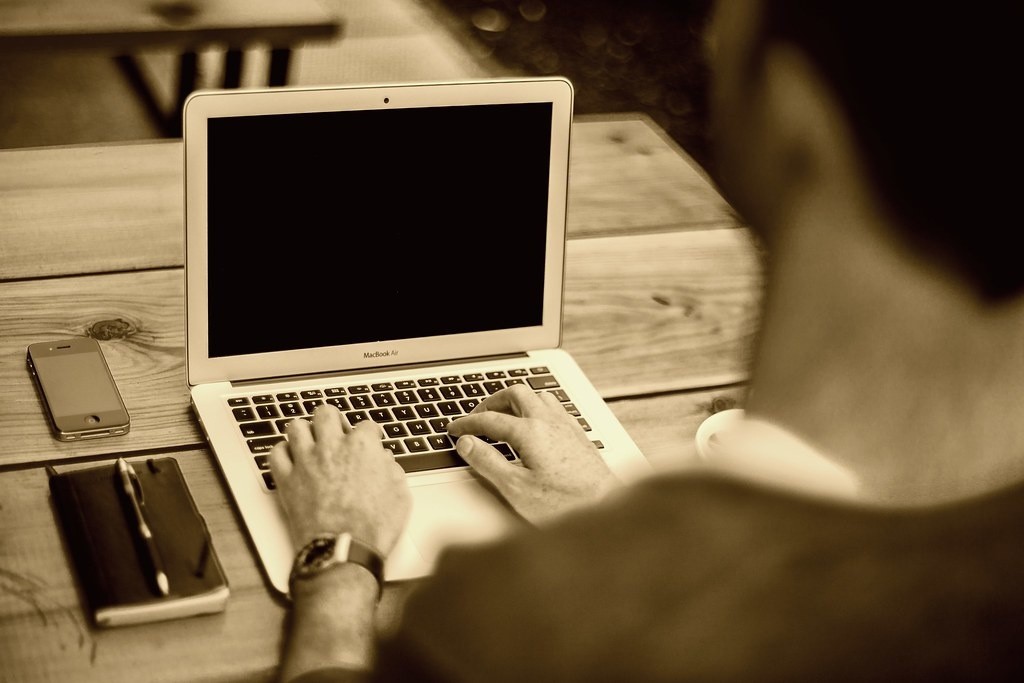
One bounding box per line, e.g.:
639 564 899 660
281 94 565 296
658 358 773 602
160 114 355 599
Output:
27 337 131 441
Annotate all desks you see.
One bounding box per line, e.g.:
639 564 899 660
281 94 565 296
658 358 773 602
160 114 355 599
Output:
0 109 760 683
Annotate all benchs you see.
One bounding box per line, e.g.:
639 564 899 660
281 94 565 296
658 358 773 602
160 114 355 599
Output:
0 0 345 142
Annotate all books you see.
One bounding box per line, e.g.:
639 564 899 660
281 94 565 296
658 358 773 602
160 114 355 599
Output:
48 456 230 628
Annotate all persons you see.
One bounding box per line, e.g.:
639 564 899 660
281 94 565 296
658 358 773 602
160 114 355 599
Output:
268 0 1024 683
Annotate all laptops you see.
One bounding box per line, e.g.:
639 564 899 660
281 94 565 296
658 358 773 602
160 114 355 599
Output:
182 76 662 600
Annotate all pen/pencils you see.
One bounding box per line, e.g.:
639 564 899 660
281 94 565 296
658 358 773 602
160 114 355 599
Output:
116 456 169 597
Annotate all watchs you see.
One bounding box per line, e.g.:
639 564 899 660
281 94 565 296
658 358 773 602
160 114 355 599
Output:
288 533 385 602
